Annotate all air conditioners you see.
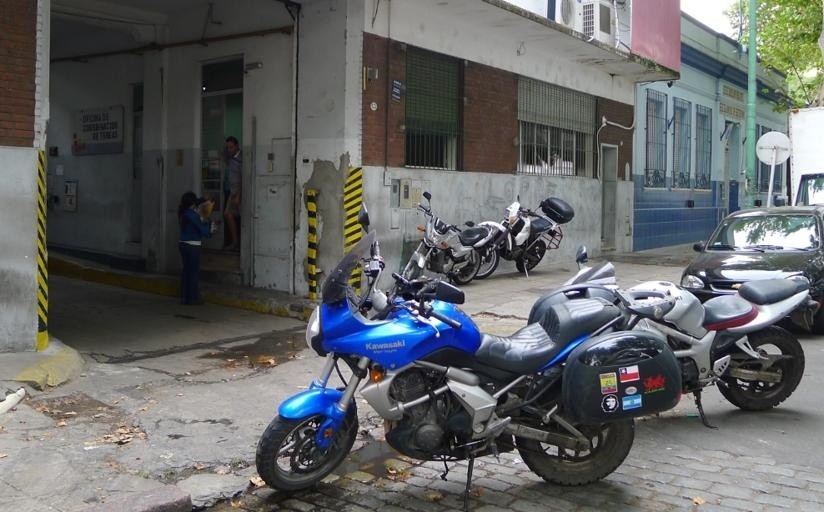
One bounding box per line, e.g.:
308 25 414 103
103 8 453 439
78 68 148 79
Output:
581 1 615 48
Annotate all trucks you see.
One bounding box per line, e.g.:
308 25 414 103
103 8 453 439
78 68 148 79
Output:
786 107 823 205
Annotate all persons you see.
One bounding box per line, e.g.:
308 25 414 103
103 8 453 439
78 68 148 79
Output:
223 136 242 252
178 192 217 306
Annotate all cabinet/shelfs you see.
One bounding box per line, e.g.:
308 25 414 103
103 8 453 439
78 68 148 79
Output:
201 156 224 251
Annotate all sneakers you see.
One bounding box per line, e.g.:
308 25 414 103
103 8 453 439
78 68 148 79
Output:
181 297 205 305
225 242 240 251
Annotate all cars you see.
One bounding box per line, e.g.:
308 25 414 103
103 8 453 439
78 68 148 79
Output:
679 205 824 335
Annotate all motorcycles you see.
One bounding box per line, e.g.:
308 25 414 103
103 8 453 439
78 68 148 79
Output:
475 195 574 277
401 191 493 285
562 243 815 429
255 201 635 494
